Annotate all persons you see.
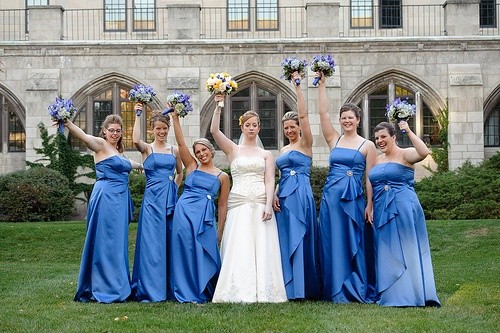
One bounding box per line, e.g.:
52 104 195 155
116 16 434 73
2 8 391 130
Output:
209 95 288 304
167 102 230 304
314 71 377 302
63 114 144 304
367 121 441 307
273 71 318 301
131 103 183 304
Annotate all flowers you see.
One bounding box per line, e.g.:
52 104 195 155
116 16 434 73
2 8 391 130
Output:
280 56 308 85
205 71 238 108
310 54 336 86
160 92 193 118
384 97 416 134
49 94 78 134
239 116 243 126
128 84 157 116
